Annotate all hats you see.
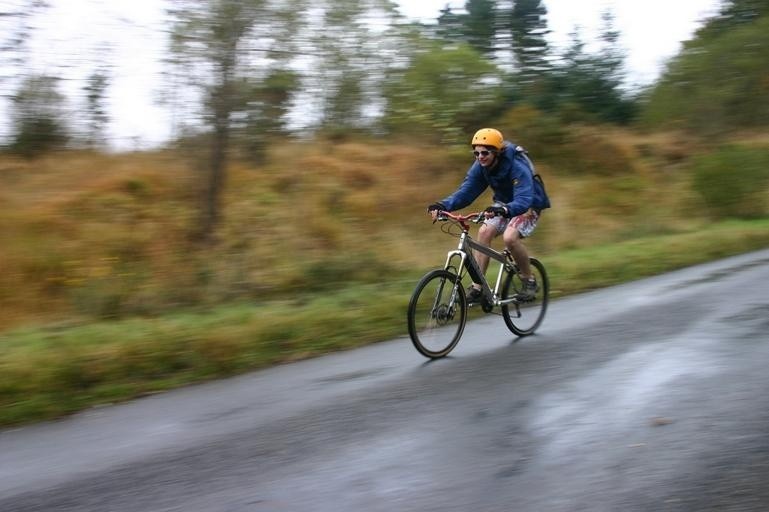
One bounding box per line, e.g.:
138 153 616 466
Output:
471 127 505 151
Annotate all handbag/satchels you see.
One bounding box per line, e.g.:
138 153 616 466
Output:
515 145 551 210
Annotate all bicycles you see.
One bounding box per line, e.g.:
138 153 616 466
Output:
407 206 549 359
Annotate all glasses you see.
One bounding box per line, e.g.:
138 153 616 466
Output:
473 151 489 156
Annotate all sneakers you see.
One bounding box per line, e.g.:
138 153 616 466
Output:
516 276 540 302
456 282 483 304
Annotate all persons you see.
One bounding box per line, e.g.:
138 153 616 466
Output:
426 128 552 305
